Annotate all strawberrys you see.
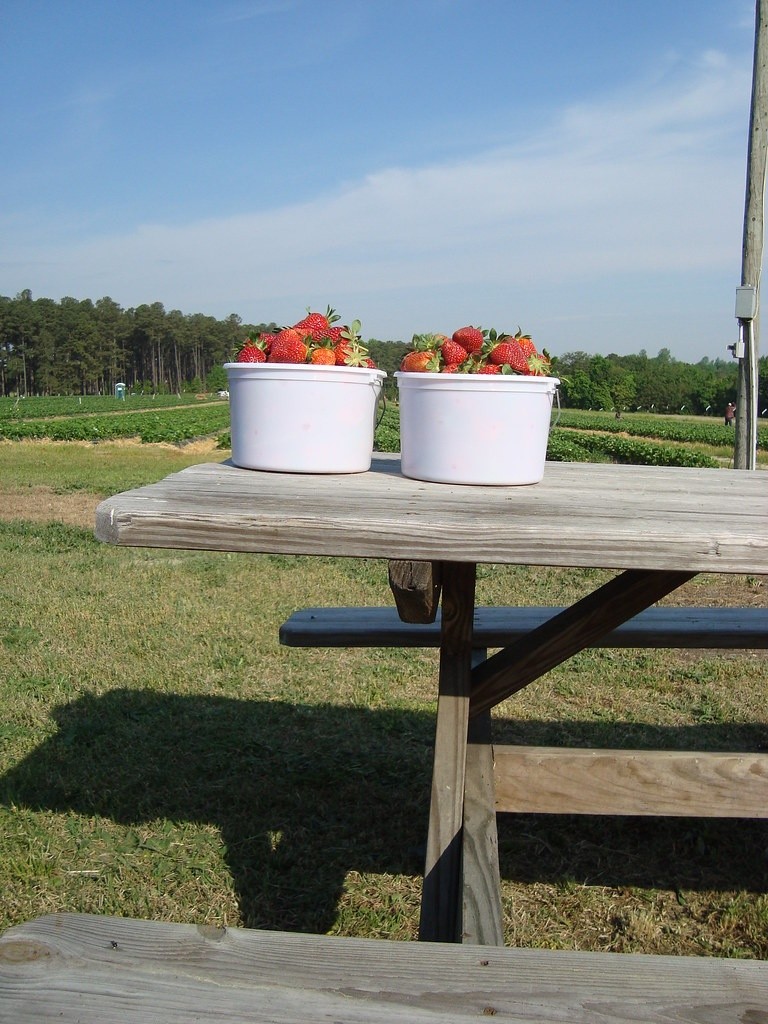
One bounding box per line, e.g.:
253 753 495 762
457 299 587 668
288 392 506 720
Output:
398 327 550 378
234 313 378 369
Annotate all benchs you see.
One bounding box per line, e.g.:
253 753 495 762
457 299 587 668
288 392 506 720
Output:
0 911 768 1024
276 606 768 650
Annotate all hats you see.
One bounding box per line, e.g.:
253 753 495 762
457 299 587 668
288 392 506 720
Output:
728 403 732 406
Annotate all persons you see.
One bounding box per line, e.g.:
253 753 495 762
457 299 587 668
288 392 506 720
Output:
615 410 623 420
725 402 736 426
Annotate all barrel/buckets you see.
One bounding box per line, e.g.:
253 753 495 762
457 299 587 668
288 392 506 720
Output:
393 369 561 487
221 359 389 475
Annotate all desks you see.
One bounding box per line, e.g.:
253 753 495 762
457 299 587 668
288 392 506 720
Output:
94 459 768 947
